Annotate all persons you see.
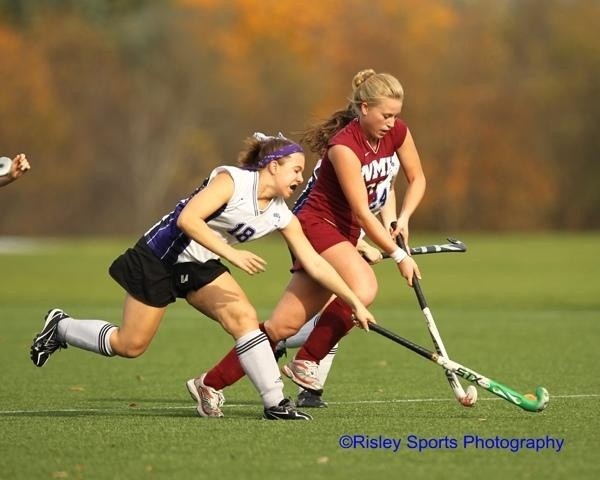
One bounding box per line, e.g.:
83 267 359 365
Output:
0 153 30 188
271 101 397 408
29 133 377 421
185 68 427 420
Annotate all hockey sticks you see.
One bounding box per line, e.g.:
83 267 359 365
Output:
362 237 467 263
392 221 477 406
367 322 548 413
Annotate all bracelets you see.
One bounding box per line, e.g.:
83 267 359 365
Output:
389 246 406 264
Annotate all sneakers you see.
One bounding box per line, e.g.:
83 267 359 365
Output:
29 307 71 367
187 372 227 418
262 398 313 420
273 337 329 408
